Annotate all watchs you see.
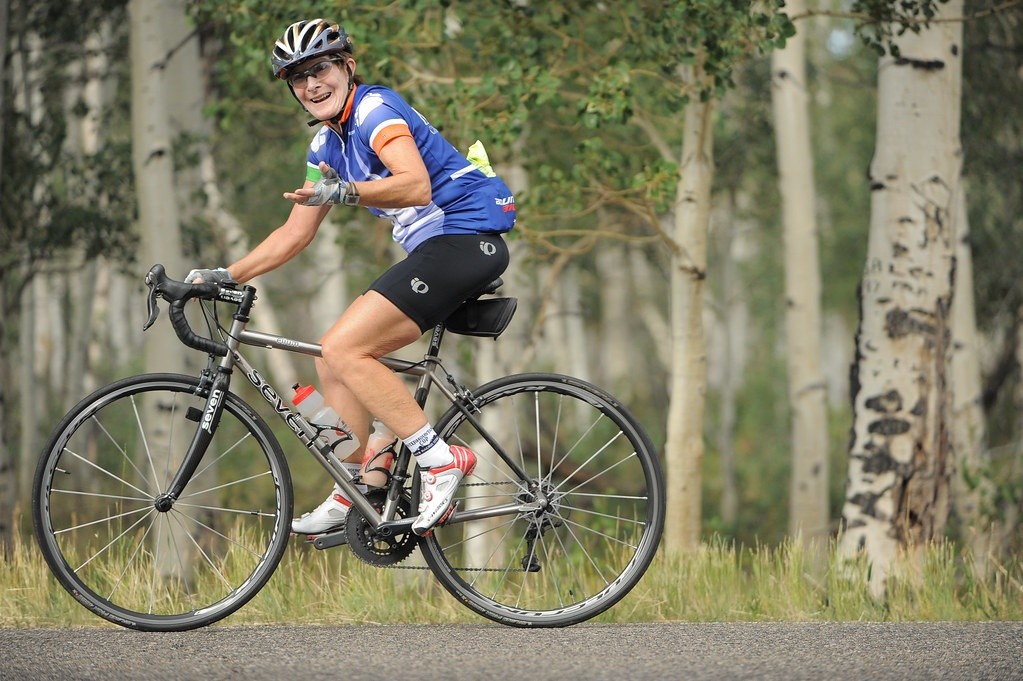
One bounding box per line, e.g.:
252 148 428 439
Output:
343 181 360 206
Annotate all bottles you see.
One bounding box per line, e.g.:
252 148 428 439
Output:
291 382 361 462
360 410 398 486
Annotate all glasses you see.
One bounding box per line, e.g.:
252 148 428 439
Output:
285 57 348 88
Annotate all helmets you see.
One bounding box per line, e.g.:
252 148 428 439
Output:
271 17 355 79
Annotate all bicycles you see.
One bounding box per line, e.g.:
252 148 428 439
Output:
30 262 668 635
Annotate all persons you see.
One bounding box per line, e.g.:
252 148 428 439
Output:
185 17 516 545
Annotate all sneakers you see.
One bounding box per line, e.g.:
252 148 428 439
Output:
291 481 368 535
411 445 477 538
